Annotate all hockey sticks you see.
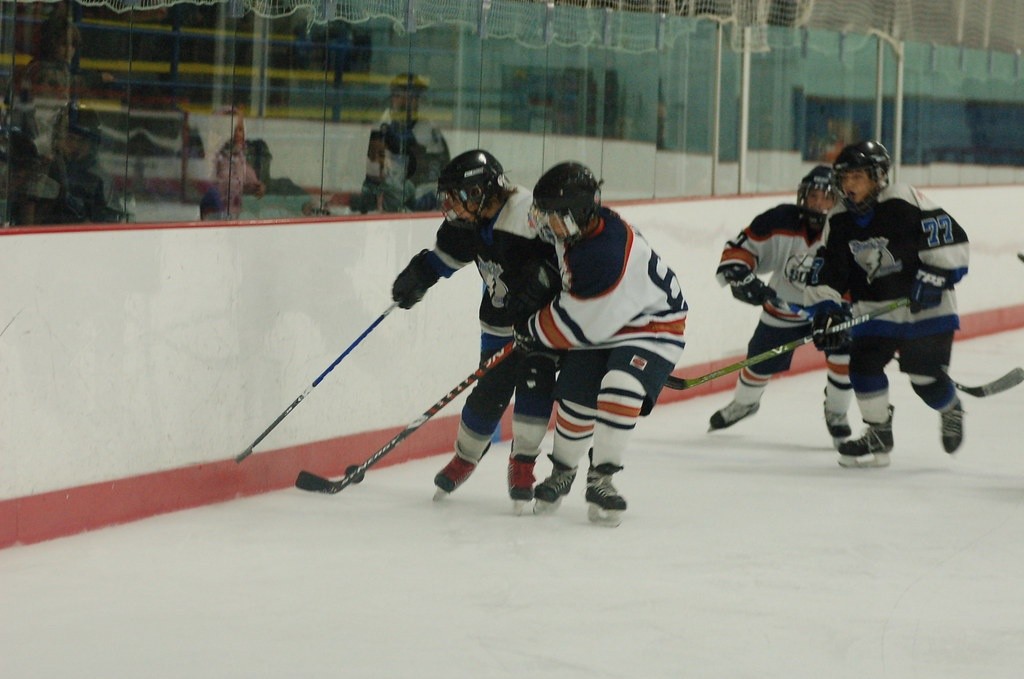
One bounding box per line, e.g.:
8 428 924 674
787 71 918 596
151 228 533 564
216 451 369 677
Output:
295 340 517 494
665 297 912 390
236 302 399 464
766 297 1024 398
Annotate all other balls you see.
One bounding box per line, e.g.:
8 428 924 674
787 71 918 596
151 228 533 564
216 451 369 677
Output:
345 465 366 483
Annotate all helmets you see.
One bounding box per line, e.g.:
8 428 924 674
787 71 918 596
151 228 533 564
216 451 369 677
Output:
833 139 891 193
795 165 838 204
391 72 427 95
50 100 102 164
437 147 507 225
530 161 603 243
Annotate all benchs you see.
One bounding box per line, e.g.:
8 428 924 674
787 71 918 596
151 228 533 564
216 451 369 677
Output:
0 10 515 144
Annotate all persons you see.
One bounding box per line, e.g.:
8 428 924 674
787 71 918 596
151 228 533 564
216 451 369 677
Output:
512 161 689 528
202 105 266 219
41 100 125 227
0 16 207 204
392 149 562 515
0 126 86 229
322 129 439 217
366 72 451 181
709 165 853 446
803 138 970 468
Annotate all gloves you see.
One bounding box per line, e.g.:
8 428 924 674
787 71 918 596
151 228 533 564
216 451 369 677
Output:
906 269 948 314
724 264 776 306
391 248 441 310
810 311 852 351
512 321 537 353
503 277 544 321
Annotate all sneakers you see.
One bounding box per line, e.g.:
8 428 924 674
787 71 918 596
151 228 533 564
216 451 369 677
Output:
709 399 759 429
823 387 852 445
507 438 541 511
585 446 629 528
533 453 580 516
938 398 964 456
433 440 491 501
836 404 896 470
